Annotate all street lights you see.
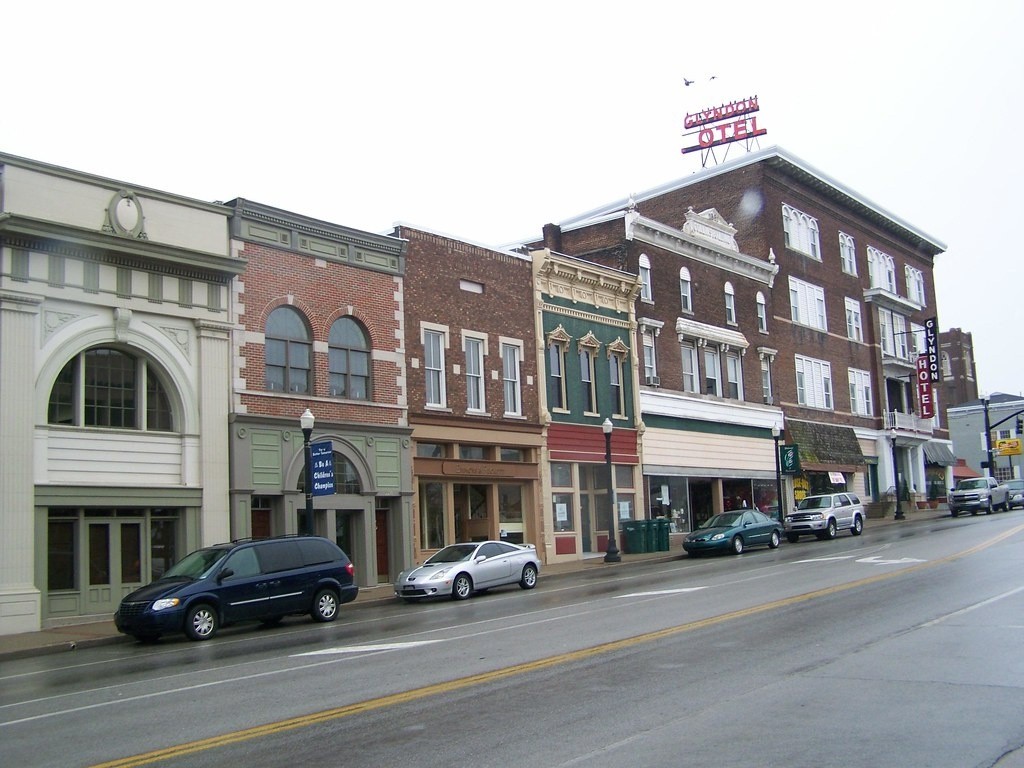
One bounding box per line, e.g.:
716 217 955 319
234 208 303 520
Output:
300 404 316 535
889 428 903 519
602 418 622 562
771 422 785 524
980 389 996 476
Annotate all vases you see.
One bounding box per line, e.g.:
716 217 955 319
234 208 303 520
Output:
916 502 928 509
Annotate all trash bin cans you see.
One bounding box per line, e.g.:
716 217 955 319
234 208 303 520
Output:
621 518 671 554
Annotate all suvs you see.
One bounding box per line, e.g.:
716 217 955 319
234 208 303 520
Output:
113 534 359 642
783 492 866 543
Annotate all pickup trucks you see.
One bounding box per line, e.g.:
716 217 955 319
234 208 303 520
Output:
948 477 1009 517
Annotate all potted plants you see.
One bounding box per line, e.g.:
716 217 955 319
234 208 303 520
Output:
927 479 939 509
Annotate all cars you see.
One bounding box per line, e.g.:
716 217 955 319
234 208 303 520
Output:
1001 479 1024 510
682 507 784 554
394 540 542 600
